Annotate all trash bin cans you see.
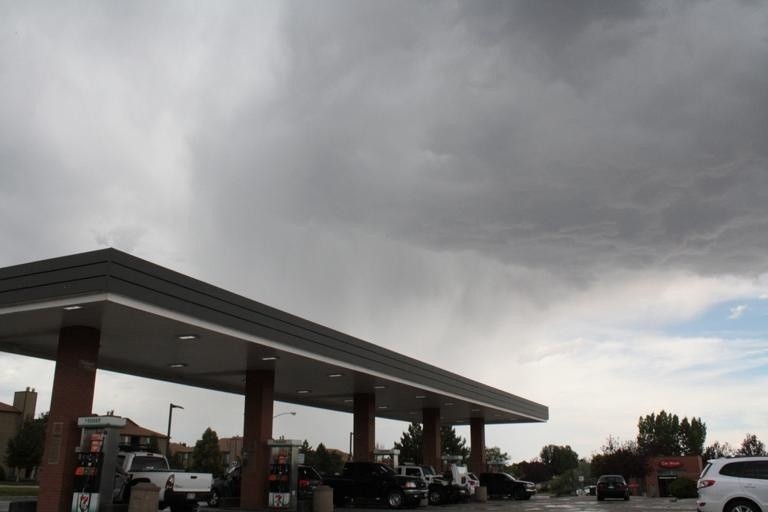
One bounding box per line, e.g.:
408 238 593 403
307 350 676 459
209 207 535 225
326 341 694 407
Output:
312 484 334 512
126 482 162 512
476 486 487 502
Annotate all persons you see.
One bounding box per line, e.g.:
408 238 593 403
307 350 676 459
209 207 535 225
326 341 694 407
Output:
113 448 129 490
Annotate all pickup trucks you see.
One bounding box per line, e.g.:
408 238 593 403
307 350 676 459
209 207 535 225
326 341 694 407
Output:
114 450 213 512
322 461 429 509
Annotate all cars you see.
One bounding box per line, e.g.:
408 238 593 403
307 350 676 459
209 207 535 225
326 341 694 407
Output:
695 456 767 512
398 462 537 505
208 462 325 507
596 474 630 501
